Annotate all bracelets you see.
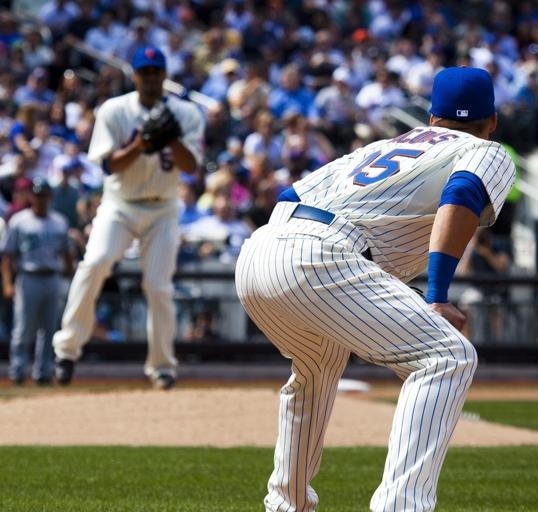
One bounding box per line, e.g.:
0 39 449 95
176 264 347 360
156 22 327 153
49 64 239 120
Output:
426 250 460 304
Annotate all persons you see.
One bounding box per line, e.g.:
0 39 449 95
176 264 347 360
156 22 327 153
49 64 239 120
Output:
230 64 518 511
51 43 208 391
0 0 538 348
2 176 76 385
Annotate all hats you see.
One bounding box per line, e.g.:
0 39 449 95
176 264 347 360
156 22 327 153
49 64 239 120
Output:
131 43 169 70
428 63 497 121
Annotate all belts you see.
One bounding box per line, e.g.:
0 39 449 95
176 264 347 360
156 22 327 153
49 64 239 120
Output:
289 203 373 262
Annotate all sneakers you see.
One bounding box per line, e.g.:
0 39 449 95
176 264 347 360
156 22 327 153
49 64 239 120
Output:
52 353 76 387
152 372 174 390
